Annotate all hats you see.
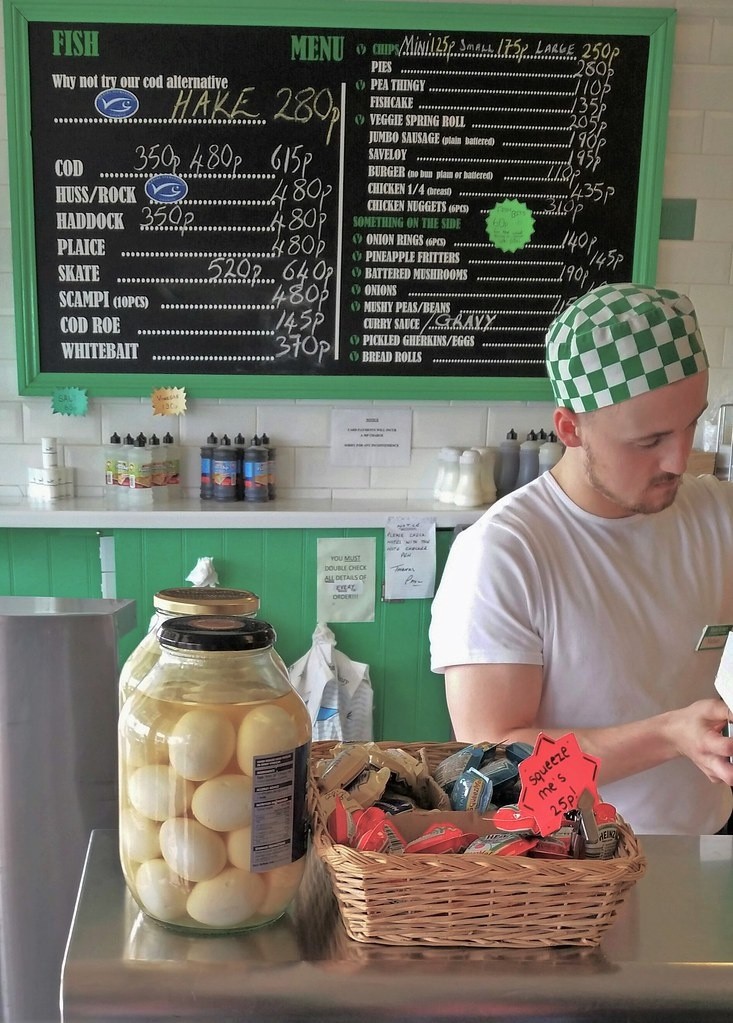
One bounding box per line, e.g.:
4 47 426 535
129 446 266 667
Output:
544 283 708 414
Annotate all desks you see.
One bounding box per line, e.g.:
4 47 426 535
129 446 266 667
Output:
1 499 490 534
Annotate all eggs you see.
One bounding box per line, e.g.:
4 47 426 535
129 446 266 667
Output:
117 685 309 927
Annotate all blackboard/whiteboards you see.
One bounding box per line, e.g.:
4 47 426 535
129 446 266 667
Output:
4 1 676 403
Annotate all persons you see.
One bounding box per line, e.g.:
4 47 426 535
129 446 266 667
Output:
431 284 733 836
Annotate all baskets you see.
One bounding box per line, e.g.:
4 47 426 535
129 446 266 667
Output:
306 741 646 951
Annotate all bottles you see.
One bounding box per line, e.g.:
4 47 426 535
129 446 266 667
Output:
198 433 277 504
432 427 564 507
102 432 184 506
